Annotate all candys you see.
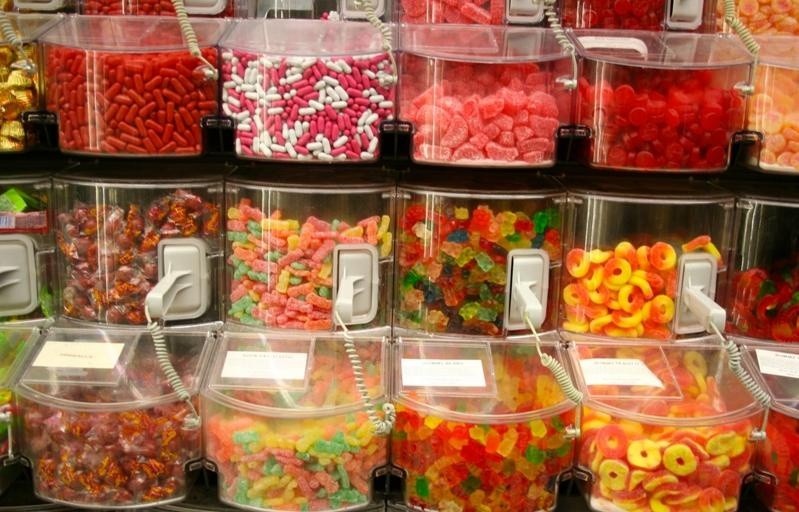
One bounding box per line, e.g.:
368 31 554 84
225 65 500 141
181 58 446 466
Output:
563 234 724 341
396 203 562 337
755 392 799 511
55 189 220 325
718 0 799 170
45 0 220 157
205 346 387 511
579 345 754 512
23 354 202 503
561 1 744 170
227 198 391 331
396 0 560 164
221 9 398 162
0 328 33 455
391 354 576 512
0 0 42 152
717 266 799 343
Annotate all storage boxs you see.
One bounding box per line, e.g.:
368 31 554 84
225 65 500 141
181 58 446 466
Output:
739 343 799 512
559 187 738 343
15 327 214 510
724 197 799 347
391 182 568 341
0 324 43 468
567 338 768 512
202 332 390 512
389 336 577 511
38 16 226 158
222 176 397 336
568 28 753 172
0 12 65 152
730 33 799 174
398 22 576 167
52 178 225 325
1 178 54 321
218 18 397 163
